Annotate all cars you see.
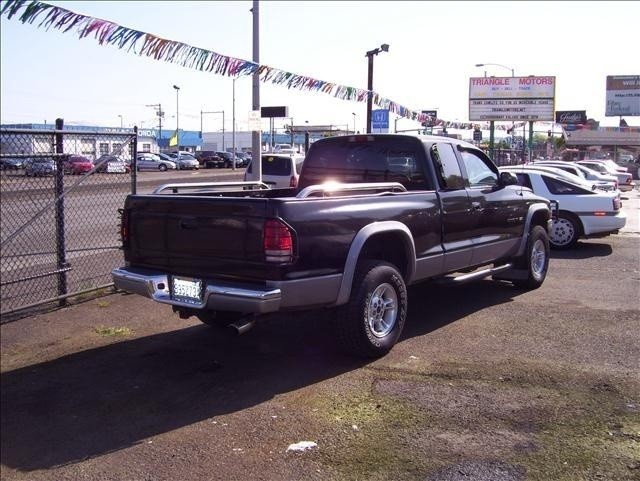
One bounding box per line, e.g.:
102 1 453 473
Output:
496 153 639 252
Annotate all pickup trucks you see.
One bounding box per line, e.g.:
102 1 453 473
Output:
109 129 559 355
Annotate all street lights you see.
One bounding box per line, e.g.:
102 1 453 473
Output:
353 113 356 134
474 62 515 78
173 85 181 170
363 42 391 132
